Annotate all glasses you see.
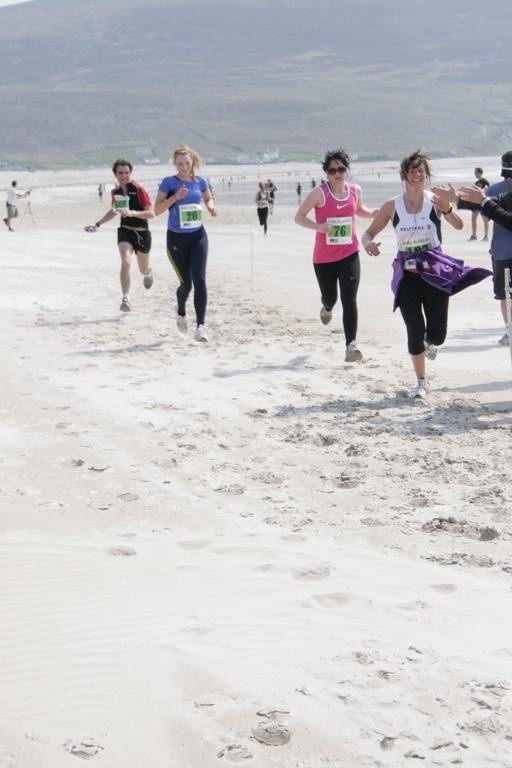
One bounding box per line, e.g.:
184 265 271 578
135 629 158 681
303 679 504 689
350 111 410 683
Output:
327 166 347 176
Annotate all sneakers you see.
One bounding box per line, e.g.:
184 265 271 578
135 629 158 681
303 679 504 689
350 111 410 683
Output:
344 343 362 363
497 333 512 346
119 297 131 312
193 323 209 344
465 233 490 244
143 270 153 290
424 326 438 361
412 378 431 397
176 313 188 334
320 305 333 326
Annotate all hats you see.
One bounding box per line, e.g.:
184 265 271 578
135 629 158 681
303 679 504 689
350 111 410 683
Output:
500 152 512 179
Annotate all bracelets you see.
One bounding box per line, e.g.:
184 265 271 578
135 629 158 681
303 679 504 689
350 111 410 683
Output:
174 194 178 201
95 222 99 228
131 210 135 218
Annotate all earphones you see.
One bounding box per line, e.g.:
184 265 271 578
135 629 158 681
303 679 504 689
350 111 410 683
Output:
426 175 427 178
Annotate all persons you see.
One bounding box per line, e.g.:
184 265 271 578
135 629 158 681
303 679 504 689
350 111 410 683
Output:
98 184 103 196
255 179 278 234
362 149 511 399
83 158 153 313
294 149 380 362
2 180 26 231
153 144 218 342
